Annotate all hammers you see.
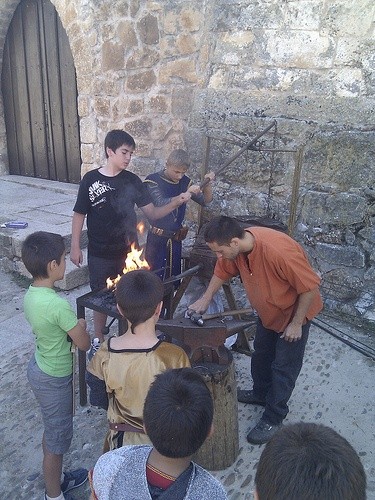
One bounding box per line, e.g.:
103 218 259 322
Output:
187 309 254 326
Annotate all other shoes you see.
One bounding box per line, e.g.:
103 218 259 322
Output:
237 389 265 406
247 420 283 444
44 491 66 500
60 468 89 493
86 337 104 364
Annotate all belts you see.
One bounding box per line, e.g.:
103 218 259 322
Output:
145 218 175 239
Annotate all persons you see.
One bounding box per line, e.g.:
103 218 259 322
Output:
88 367 227 500
22 231 91 500
255 422 368 500
70 130 192 357
145 148 215 317
188 215 323 445
86 270 191 451
